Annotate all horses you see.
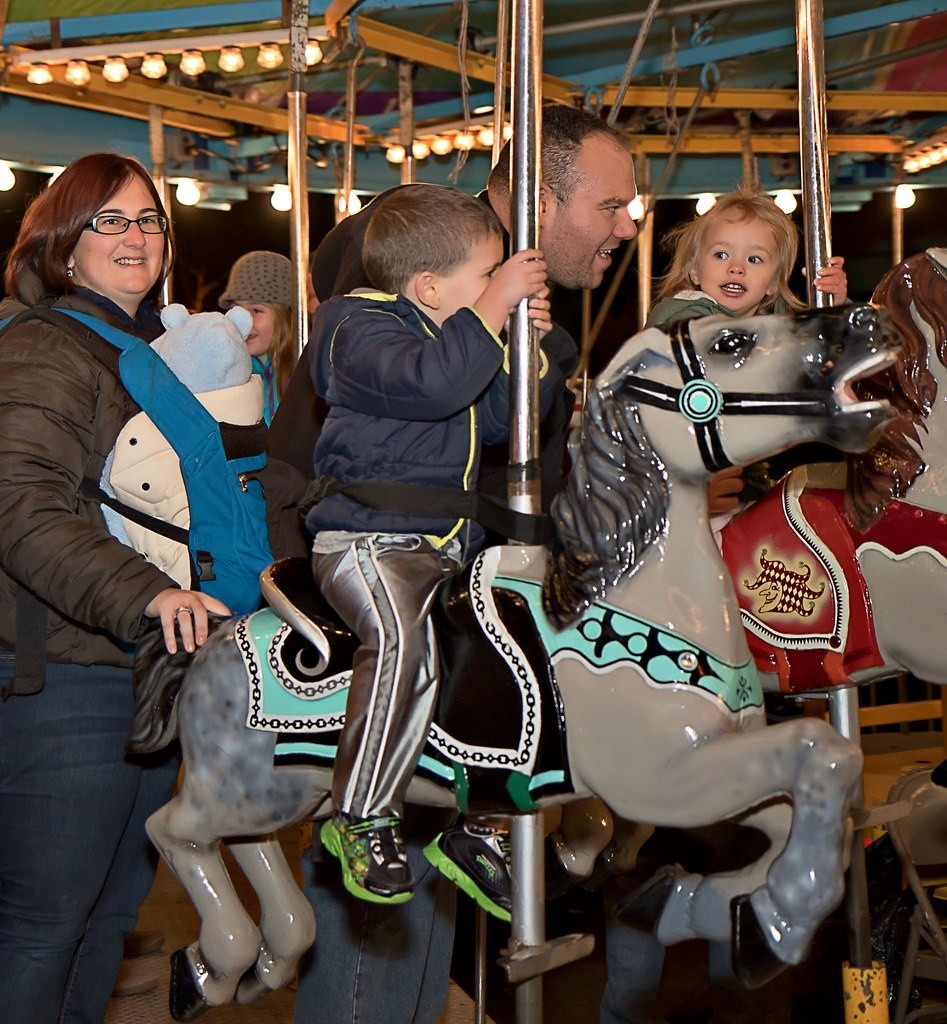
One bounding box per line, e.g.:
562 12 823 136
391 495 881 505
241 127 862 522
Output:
146 297 906 1017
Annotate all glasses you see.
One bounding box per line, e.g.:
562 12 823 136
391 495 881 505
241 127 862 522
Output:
83 215 168 234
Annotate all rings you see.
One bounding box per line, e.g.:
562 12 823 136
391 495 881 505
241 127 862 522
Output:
175 605 190 616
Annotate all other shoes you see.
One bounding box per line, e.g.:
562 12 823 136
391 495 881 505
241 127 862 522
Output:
646 821 769 874
111 962 159 996
123 929 166 959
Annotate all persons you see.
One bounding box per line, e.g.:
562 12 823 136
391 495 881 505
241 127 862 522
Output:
598 914 733 1024
0 151 235 1024
259 103 643 1024
643 176 853 330
217 250 320 430
97 303 276 618
302 184 555 924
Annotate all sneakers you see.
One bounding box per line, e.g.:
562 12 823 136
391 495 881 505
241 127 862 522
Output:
421 821 514 921
321 811 415 904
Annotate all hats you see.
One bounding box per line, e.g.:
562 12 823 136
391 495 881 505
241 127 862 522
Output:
218 251 292 306
